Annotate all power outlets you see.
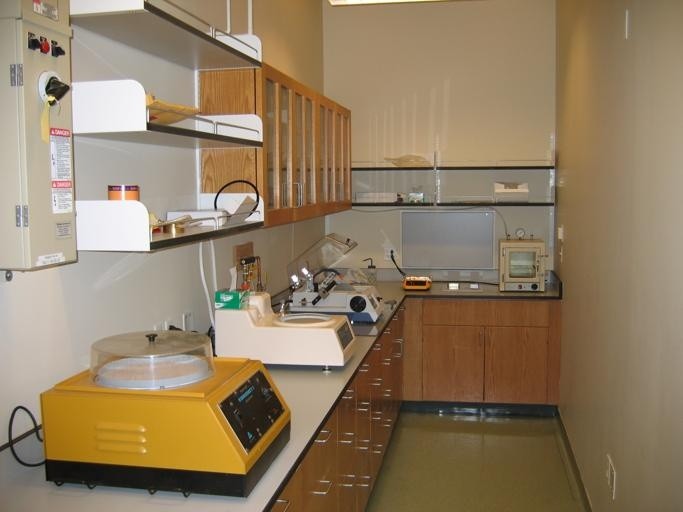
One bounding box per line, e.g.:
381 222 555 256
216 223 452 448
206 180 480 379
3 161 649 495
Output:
385 247 397 262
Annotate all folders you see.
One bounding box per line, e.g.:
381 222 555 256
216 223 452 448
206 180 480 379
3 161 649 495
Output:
355 192 403 203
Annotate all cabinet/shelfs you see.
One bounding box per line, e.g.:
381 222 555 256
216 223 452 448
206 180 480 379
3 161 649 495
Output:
271 280 403 512
69 0 264 254
200 62 351 229
403 281 559 419
